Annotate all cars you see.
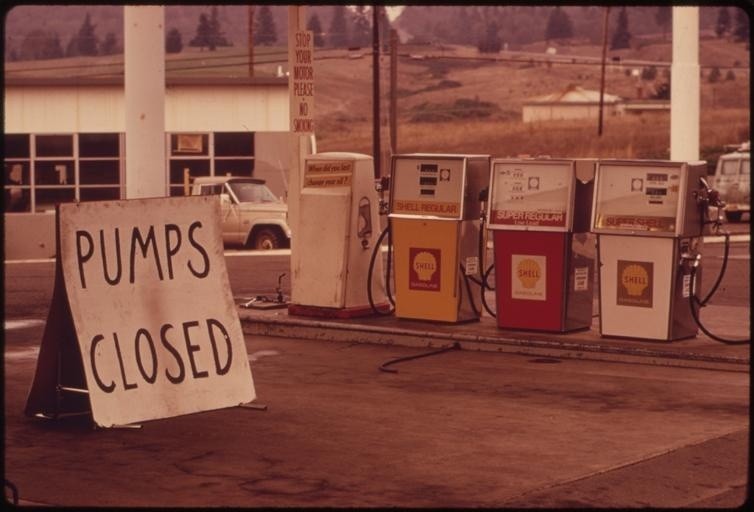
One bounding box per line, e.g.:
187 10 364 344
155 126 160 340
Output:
713 147 752 222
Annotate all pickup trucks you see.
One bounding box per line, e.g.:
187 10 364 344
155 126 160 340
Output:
179 176 292 250
